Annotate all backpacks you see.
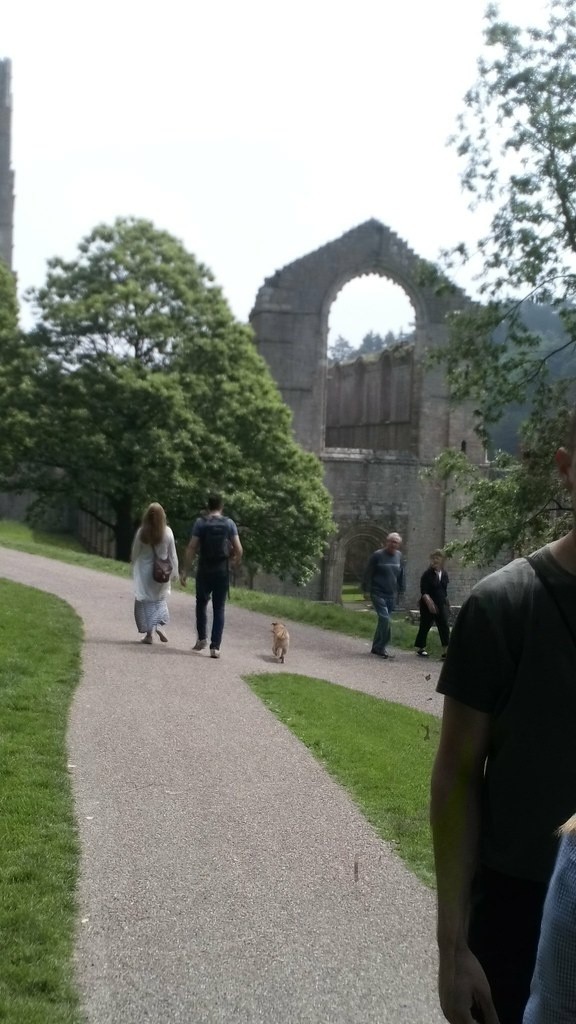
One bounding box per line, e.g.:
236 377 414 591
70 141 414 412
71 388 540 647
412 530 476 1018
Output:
200 514 231 560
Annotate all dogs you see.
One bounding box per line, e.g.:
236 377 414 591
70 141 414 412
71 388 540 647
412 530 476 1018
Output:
271 622 290 664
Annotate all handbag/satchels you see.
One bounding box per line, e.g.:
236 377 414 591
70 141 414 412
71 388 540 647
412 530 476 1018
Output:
153 555 173 583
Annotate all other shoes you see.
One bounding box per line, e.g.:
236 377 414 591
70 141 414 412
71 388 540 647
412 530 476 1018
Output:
155 623 169 642
417 648 428 656
441 653 448 661
210 649 221 658
371 647 397 659
192 639 208 651
141 635 153 644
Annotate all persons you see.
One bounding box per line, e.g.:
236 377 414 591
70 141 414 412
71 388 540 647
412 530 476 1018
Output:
180 494 243 658
522 812 576 1024
361 532 405 658
415 549 450 659
430 428 576 1024
130 502 178 644
